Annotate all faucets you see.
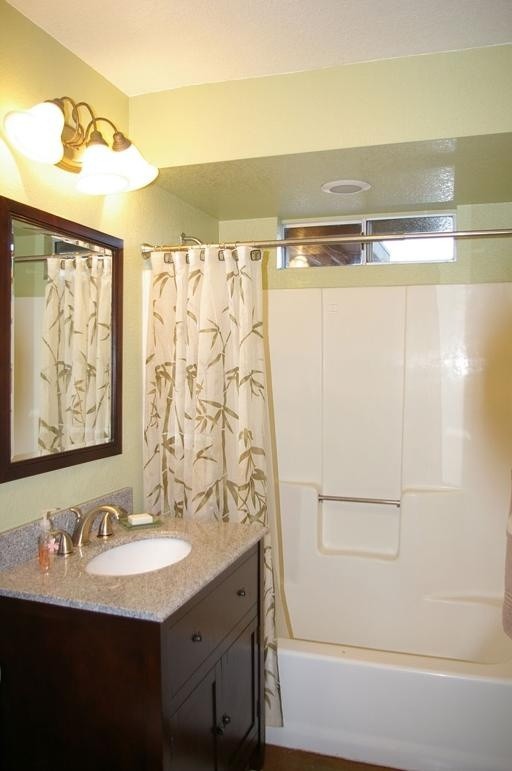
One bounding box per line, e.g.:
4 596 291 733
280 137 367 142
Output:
69 500 121 548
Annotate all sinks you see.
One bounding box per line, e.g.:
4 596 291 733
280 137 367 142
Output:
82 531 194 578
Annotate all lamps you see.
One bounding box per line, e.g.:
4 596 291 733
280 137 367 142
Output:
2 96 158 196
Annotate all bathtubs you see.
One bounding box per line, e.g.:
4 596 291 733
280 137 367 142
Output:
265 585 512 769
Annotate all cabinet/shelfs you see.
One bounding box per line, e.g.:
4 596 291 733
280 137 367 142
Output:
0 538 266 771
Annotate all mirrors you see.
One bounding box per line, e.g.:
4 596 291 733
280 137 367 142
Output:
0 199 123 484
